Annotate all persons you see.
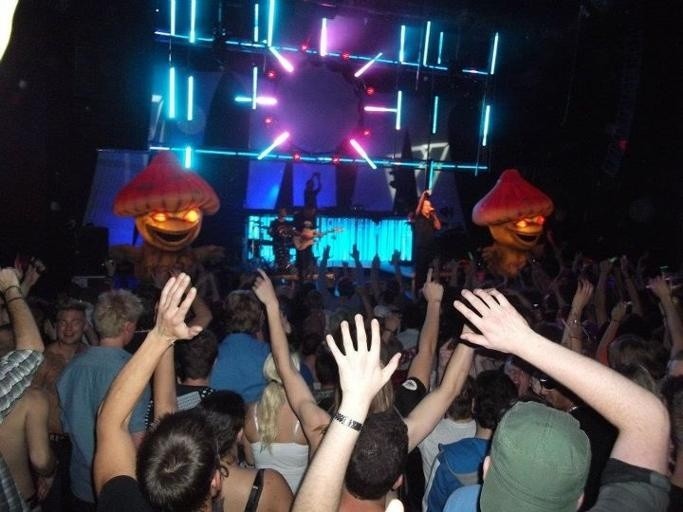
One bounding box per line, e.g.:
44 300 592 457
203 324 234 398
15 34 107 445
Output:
413 190 441 288
304 173 322 208
1 246 683 510
268 207 295 273
289 203 321 270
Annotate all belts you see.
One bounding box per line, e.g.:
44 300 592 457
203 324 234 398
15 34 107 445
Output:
49 433 65 441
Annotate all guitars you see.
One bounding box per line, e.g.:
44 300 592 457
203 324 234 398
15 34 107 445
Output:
294 226 341 251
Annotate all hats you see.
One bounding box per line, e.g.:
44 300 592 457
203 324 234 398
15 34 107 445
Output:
479 401 592 511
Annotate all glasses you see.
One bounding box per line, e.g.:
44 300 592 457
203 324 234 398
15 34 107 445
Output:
57 318 82 327
